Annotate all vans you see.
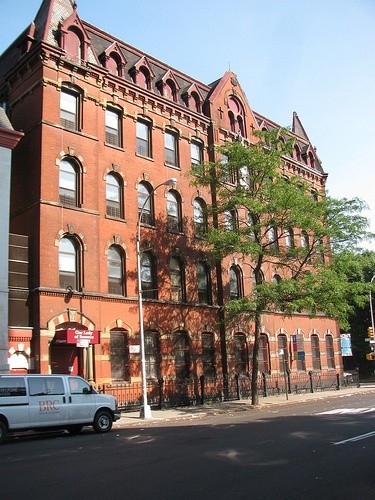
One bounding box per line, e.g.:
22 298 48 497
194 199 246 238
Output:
0 374 123 442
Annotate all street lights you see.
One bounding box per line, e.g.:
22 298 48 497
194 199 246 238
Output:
134 177 178 419
